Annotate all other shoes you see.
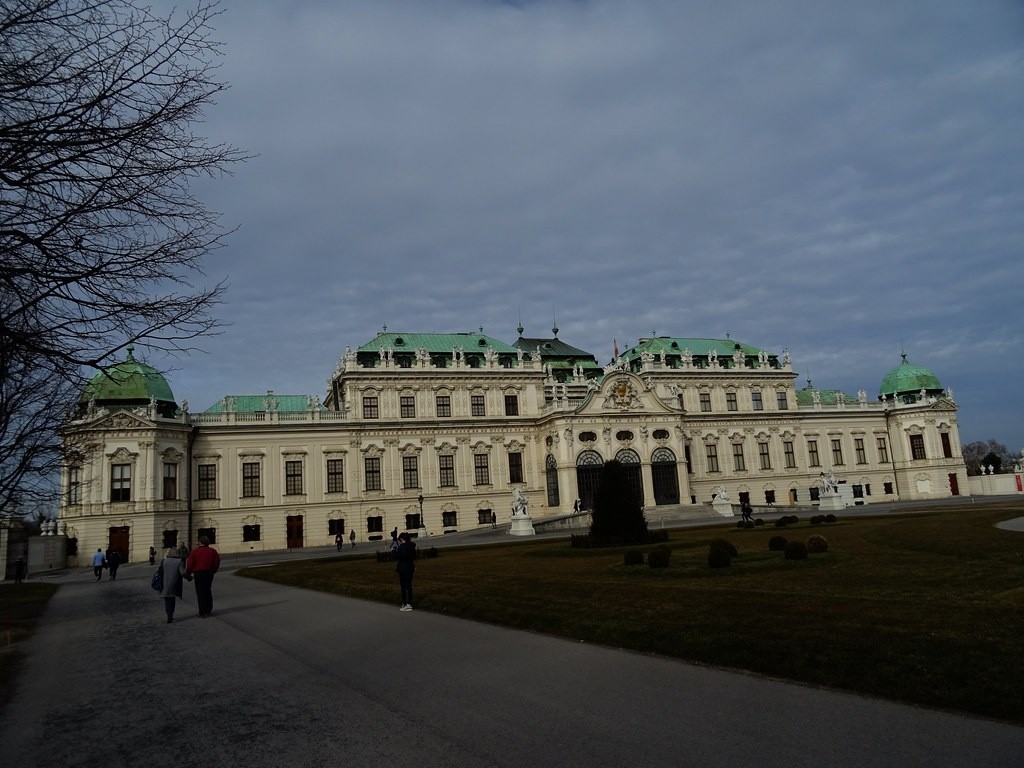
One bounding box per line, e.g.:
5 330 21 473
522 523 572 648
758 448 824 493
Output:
96 576 99 581
109 576 111 581
196 613 204 618
98 580 101 583
167 618 172 623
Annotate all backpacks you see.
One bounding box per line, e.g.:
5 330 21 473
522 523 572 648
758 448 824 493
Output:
337 536 341 541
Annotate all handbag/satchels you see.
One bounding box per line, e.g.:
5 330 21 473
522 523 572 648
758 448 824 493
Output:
151 559 166 591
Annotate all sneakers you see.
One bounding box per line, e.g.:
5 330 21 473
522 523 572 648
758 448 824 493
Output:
400 604 413 612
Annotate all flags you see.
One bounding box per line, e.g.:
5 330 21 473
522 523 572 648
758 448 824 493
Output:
614 339 619 357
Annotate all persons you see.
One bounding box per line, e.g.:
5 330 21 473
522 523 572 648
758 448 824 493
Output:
335 532 343 551
491 512 497 529
573 499 581 515
106 547 119 581
390 527 400 549
741 502 755 521
767 495 773 505
149 547 156 565
15 557 24 583
350 530 356 548
178 542 189 568
389 532 416 611
186 535 221 618
159 548 192 622
93 548 108 582
